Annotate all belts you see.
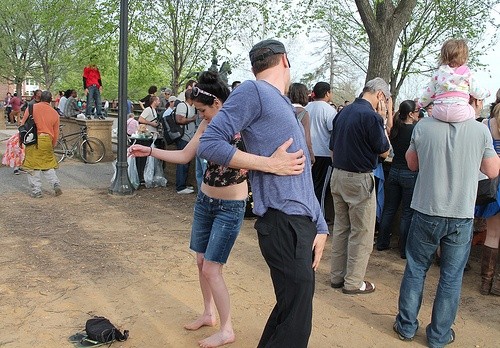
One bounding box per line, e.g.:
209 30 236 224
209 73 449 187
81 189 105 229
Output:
335 167 373 173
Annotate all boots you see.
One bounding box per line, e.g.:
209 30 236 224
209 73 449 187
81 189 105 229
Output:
480 244 500 296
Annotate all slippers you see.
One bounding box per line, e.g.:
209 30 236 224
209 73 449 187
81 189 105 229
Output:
68 329 116 348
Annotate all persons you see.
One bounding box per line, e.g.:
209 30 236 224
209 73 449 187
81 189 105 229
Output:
415 38 491 123
391 94 500 348
53 89 87 119
196 39 329 348
2 132 26 175
91 99 117 118
129 71 249 348
82 54 106 120
0 89 42 126
126 77 500 297
14 90 43 175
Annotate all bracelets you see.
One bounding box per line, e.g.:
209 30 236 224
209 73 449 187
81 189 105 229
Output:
148 146 152 156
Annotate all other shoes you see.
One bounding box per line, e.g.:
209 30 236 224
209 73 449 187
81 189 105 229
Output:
86 116 91 120
54 183 63 195
342 281 375 294
331 281 344 288
177 186 195 195
393 321 414 342
447 328 455 344
15 170 19 174
97 116 105 120
31 190 43 198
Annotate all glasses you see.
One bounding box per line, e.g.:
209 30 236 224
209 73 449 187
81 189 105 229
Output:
192 87 217 99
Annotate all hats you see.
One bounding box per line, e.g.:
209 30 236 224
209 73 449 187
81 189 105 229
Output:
168 96 177 102
249 40 290 68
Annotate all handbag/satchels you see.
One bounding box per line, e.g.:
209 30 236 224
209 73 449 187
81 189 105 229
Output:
86 316 129 343
17 104 37 146
475 179 496 205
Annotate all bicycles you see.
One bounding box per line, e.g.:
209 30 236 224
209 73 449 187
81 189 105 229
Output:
52 121 106 164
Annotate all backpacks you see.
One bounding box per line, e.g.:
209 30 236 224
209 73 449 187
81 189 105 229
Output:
161 101 188 144
